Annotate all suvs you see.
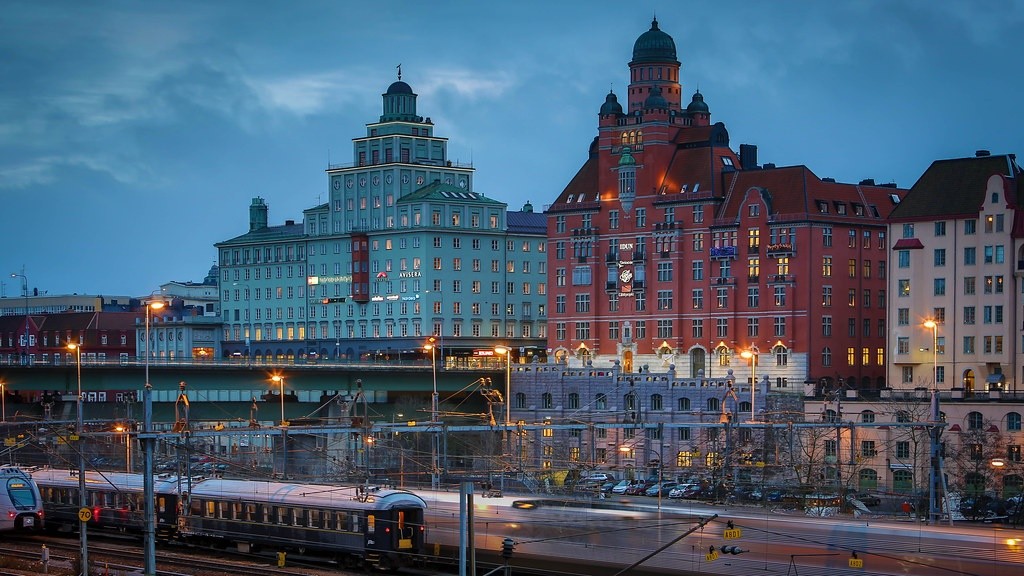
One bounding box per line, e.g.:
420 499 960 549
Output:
585 473 614 483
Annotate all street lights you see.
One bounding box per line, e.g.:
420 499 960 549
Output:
12 273 28 364
923 320 938 391
146 301 165 385
496 344 512 423
742 350 754 423
272 374 283 428
426 342 438 422
68 343 82 397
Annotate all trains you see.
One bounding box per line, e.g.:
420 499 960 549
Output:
0 466 44 534
26 465 427 572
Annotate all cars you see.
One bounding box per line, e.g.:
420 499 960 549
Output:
481 489 503 498
1007 494 1024 504
599 478 881 509
84 456 228 474
